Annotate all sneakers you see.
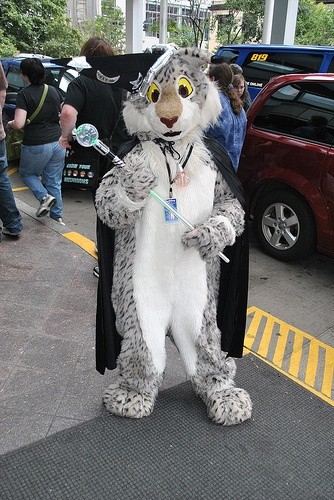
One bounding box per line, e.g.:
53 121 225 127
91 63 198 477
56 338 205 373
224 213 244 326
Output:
57 218 66 227
36 195 57 217
2 225 22 236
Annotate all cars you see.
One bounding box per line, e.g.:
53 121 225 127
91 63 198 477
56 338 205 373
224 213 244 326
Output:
1 59 81 123
237 72 334 263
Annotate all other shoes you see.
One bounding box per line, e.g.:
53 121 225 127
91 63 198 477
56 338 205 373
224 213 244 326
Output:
93 266 99 278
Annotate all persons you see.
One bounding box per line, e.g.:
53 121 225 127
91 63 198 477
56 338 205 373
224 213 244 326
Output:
200 56 250 173
9 58 65 226
59 38 126 278
0 64 23 238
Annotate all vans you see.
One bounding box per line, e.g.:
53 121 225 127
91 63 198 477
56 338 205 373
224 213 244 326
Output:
213 45 334 113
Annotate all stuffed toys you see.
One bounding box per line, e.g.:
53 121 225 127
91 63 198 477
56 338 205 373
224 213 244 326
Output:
72 48 253 425
